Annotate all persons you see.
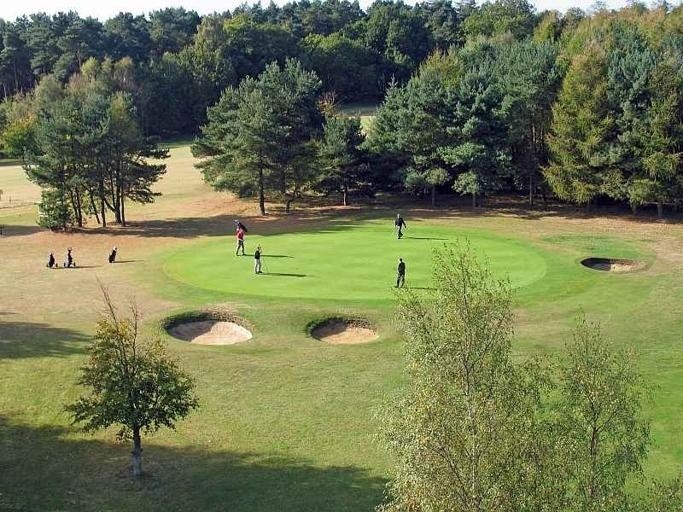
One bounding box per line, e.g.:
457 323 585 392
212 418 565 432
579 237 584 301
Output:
394 257 406 288
109 247 117 263
255 246 264 274
394 214 406 239
49 253 54 267
67 249 73 268
235 226 248 257
